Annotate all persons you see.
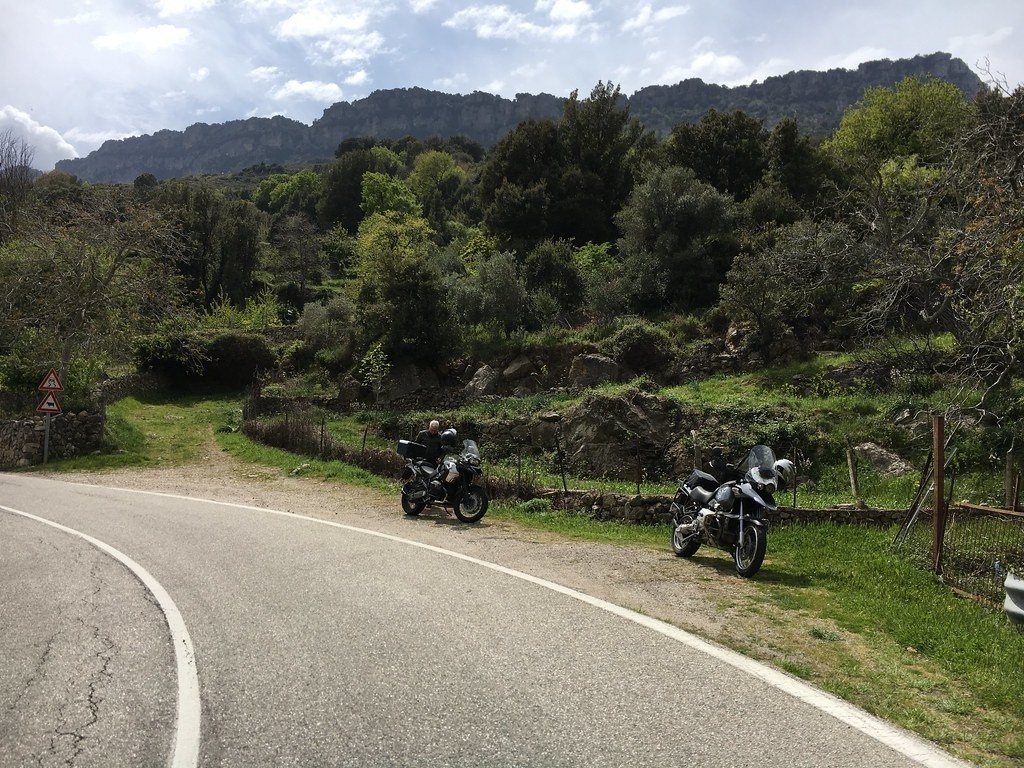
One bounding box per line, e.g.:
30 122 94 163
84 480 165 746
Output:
415 420 444 510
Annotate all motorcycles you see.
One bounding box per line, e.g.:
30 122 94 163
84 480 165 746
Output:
396 440 489 524
668 445 795 578
